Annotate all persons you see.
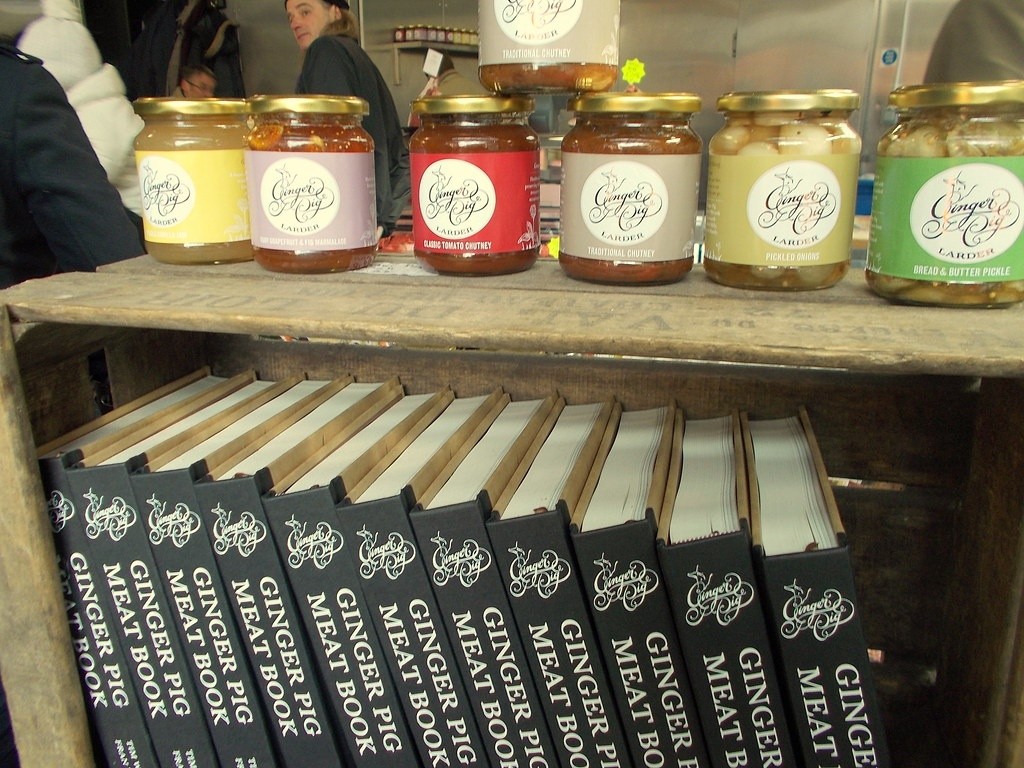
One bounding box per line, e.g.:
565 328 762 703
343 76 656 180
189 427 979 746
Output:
282 1 410 248
0 1 219 440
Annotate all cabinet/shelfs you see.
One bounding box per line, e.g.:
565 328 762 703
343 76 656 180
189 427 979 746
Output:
0 238 1024 768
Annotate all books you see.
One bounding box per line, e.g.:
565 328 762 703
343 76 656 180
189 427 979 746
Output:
38 366 892 768
